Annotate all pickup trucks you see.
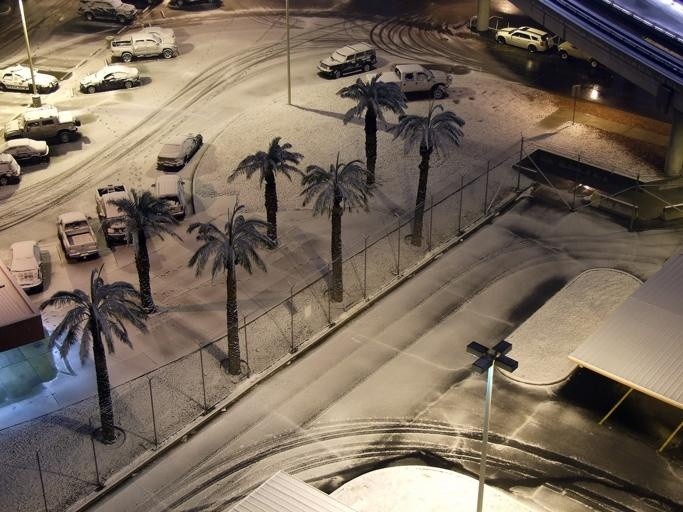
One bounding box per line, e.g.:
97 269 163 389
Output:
55 211 99 261
95 183 136 249
110 35 182 62
365 65 453 100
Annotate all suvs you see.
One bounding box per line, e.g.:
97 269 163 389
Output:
0 154 23 186
149 174 187 220
318 42 378 80
495 25 555 54
5 104 80 146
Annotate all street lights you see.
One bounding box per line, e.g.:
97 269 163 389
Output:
466 339 519 512
0 0 41 107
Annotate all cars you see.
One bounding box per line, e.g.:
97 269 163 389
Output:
0 65 60 94
170 0 222 6
76 0 139 24
8 240 44 295
0 138 49 164
558 40 599 68
81 65 141 93
126 0 156 4
121 27 177 40
156 133 205 171
